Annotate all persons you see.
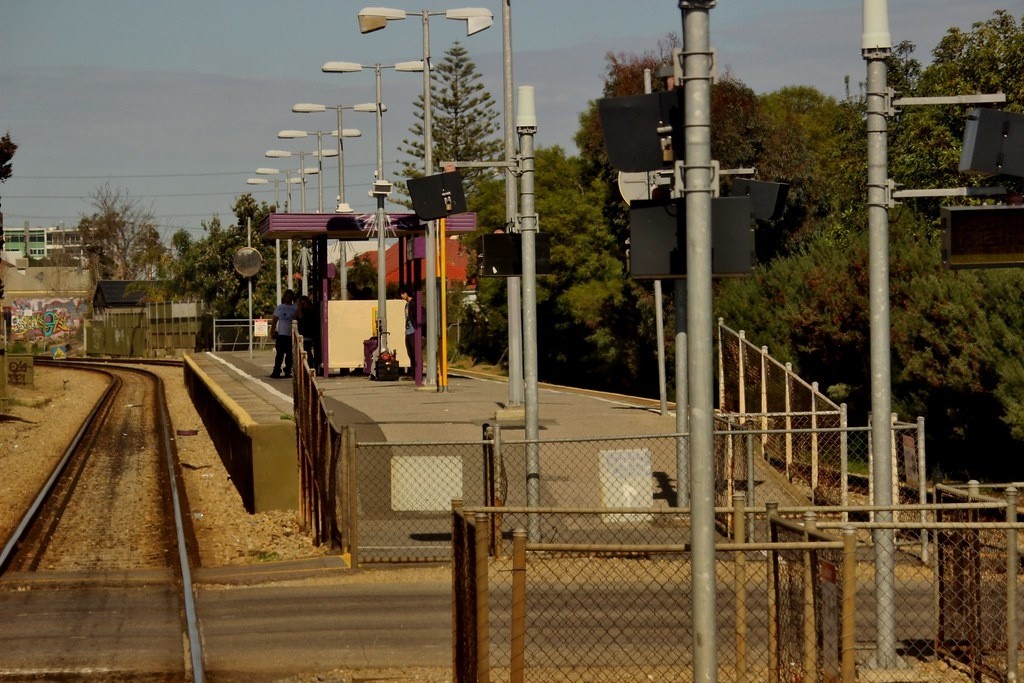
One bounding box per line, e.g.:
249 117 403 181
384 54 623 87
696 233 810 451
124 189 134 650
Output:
399 285 424 381
270 289 298 379
295 295 319 376
346 281 373 300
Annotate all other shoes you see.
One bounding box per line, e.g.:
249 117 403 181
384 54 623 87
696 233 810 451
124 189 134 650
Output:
270 373 279 378
285 373 292 377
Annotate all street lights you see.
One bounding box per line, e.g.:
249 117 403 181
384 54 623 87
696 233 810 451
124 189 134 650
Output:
246 178 307 304
278 129 362 301
292 102 388 301
256 168 319 290
358 7 494 387
321 61 430 354
265 150 338 297
